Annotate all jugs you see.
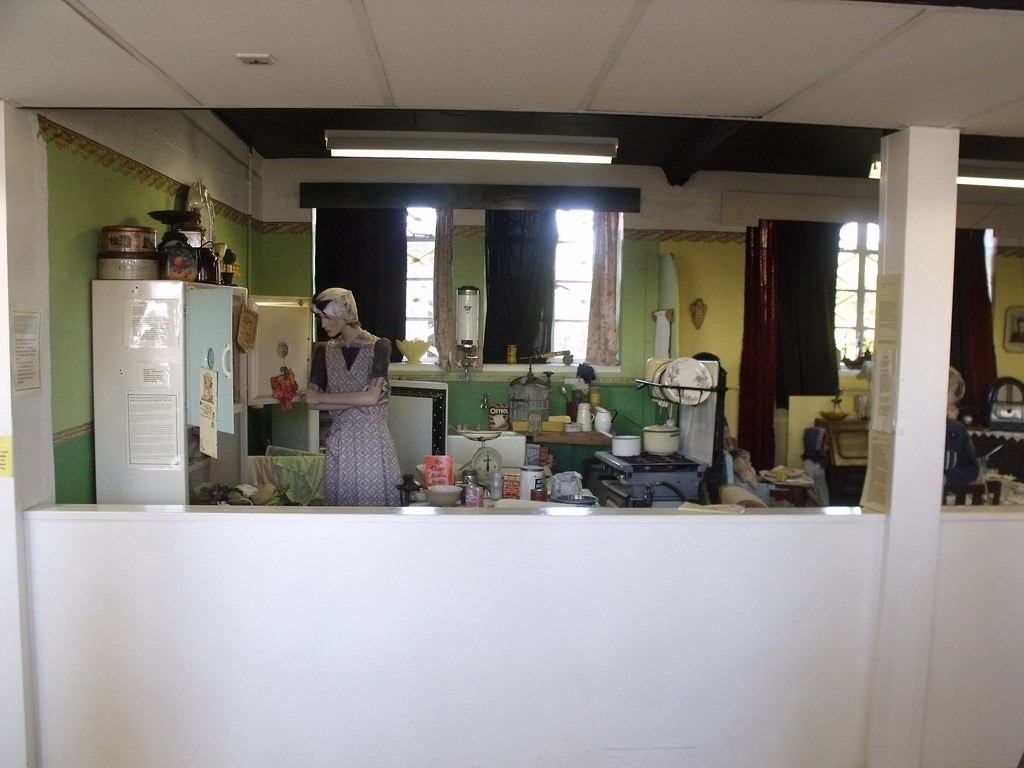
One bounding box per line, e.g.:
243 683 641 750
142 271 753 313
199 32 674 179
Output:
595 409 618 433
576 403 594 432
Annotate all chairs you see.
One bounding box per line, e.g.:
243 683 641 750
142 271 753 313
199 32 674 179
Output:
944 480 1002 505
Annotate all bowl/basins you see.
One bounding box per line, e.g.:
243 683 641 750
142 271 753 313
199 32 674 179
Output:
612 435 641 456
424 488 465 507
416 464 425 482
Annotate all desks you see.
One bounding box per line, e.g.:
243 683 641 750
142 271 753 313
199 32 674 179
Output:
524 431 616 446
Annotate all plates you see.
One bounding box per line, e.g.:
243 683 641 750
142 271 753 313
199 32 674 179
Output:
653 356 713 406
550 495 596 505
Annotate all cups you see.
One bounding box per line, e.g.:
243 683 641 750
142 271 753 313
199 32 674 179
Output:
507 345 516 365
424 456 454 486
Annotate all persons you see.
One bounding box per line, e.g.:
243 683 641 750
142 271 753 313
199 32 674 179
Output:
941 366 980 505
304 288 403 507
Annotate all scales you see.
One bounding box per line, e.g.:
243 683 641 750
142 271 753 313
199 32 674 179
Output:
147 208 201 249
460 430 505 474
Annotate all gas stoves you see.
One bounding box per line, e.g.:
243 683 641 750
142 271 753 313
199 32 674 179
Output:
595 450 709 501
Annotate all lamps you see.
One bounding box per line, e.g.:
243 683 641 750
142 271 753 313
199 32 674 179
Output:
324 129 619 165
868 153 1024 189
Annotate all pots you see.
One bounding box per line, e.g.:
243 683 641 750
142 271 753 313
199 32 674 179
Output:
644 425 680 456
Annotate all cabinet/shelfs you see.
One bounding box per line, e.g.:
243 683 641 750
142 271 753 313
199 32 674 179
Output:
968 426 1024 483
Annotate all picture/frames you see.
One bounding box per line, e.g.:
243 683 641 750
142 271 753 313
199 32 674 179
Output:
1003 304 1024 354
